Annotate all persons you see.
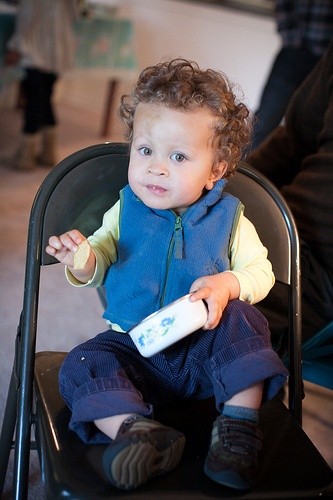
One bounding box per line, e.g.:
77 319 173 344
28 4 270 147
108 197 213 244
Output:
47 58 287 490
5 1 73 170
240 0 333 158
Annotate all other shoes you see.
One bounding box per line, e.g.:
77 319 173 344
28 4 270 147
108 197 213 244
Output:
16 145 59 169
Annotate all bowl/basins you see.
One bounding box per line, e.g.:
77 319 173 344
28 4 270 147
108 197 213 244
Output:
126 293 209 359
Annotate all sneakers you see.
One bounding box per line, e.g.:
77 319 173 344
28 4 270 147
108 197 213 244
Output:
204 416 263 489
101 416 185 488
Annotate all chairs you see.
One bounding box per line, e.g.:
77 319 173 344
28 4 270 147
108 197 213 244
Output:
0 142 332 500
